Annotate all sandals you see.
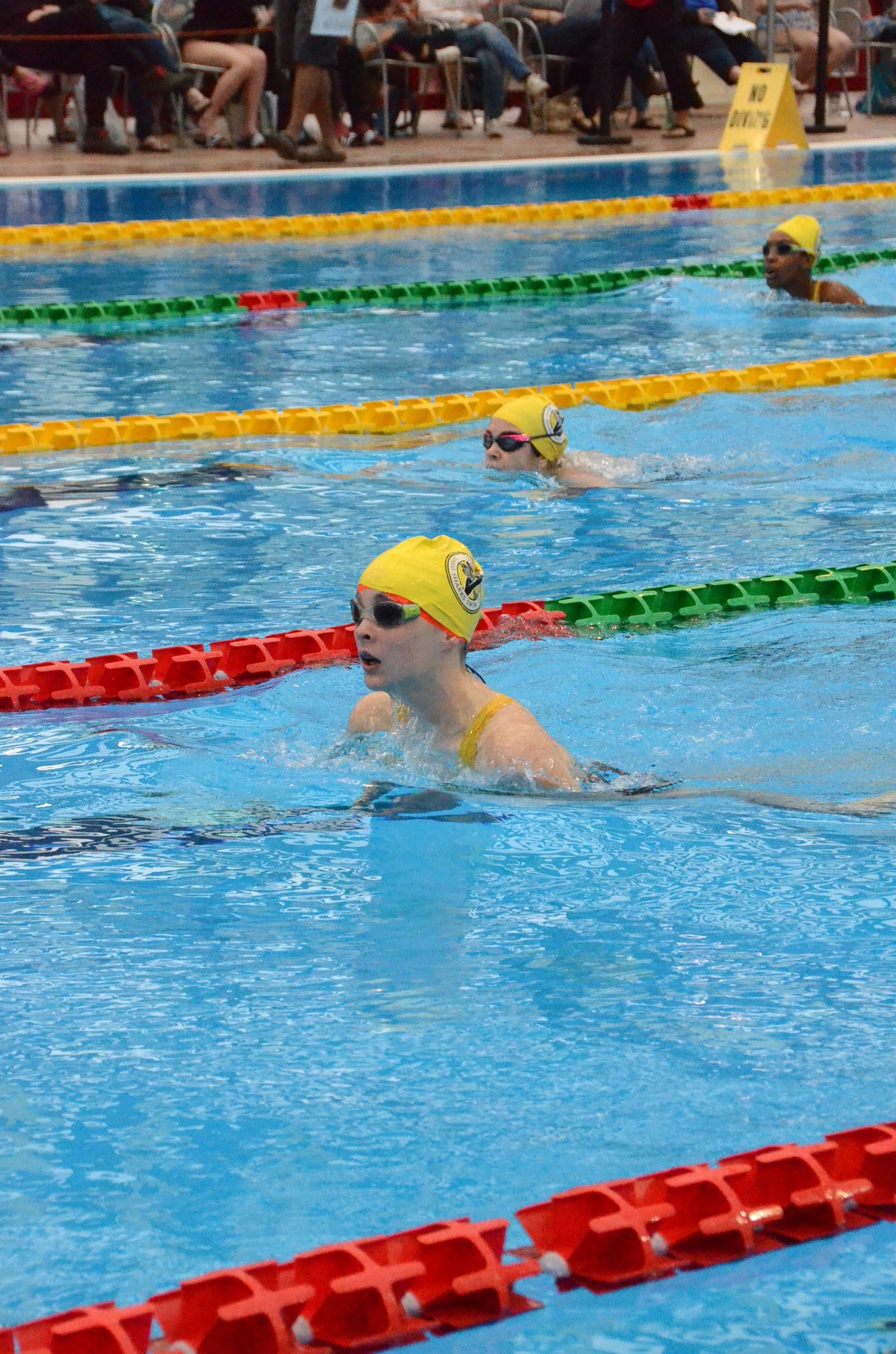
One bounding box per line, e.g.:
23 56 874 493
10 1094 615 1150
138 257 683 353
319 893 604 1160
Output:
662 124 696 138
632 118 661 129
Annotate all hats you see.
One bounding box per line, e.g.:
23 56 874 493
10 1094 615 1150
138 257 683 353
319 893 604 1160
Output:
357 535 484 642
772 214 822 258
494 394 567 464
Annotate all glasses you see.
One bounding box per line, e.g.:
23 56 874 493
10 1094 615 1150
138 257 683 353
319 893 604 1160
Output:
483 432 531 454
350 596 423 626
763 242 807 254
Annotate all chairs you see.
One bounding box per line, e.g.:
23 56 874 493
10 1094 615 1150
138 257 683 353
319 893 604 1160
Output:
2 0 896 150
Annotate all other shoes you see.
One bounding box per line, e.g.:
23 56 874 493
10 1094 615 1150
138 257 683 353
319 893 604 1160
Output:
446 112 472 128
324 141 345 161
81 129 129 154
274 132 296 159
353 124 383 146
49 129 76 143
187 94 210 113
249 132 267 147
574 114 599 133
196 130 232 149
140 136 169 152
334 124 361 147
296 129 321 161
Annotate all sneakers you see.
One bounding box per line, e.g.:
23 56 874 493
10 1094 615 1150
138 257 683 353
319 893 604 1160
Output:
486 117 503 137
523 74 549 96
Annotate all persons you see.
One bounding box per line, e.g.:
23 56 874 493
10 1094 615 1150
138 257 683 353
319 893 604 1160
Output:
762 214 866 306
483 394 614 486
346 535 584 795
0 0 850 159
